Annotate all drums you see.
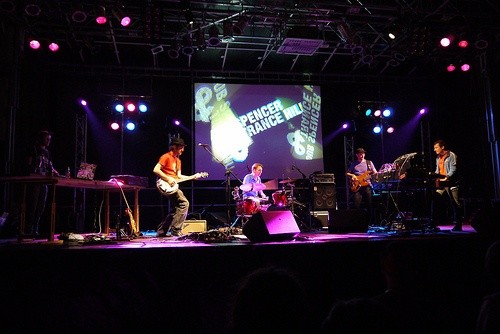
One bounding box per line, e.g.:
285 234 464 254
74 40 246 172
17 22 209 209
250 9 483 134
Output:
272 190 289 209
236 198 256 215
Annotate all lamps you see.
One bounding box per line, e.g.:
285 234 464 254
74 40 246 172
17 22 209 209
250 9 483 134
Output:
388 27 401 40
368 104 381 117
382 120 394 134
440 32 470 73
360 105 372 116
195 29 207 51
24 0 42 17
207 25 220 47
232 16 250 35
69 0 88 23
220 20 235 44
368 121 381 133
94 5 107 25
112 6 130 27
181 33 194 56
377 103 391 117
168 40 182 59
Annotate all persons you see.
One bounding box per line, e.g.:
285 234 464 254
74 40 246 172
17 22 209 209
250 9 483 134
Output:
233 240 500 334
345 148 379 208
153 140 204 237
242 163 266 198
433 139 464 231
25 131 60 239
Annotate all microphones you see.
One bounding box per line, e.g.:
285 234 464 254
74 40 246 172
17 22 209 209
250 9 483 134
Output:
198 143 208 146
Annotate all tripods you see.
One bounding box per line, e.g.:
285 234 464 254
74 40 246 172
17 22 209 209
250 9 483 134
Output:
229 190 250 230
285 182 311 234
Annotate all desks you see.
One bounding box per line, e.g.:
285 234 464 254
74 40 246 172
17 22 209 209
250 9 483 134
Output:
0 176 146 243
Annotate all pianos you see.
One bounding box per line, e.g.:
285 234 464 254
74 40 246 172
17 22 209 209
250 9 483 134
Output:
372 173 449 233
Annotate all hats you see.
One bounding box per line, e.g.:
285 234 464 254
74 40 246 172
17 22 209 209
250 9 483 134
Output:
356 147 366 153
170 139 187 146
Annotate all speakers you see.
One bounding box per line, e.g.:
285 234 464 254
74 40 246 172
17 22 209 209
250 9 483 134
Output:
328 208 369 233
240 209 301 244
309 183 336 211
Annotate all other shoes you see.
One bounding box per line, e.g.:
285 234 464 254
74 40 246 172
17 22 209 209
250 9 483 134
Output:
451 223 462 231
427 221 436 227
29 231 40 238
167 229 185 238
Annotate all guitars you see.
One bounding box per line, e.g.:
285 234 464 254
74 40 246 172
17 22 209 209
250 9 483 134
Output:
350 170 382 192
156 171 208 196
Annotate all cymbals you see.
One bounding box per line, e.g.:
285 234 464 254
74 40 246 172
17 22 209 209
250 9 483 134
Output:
279 180 295 183
239 183 266 191
